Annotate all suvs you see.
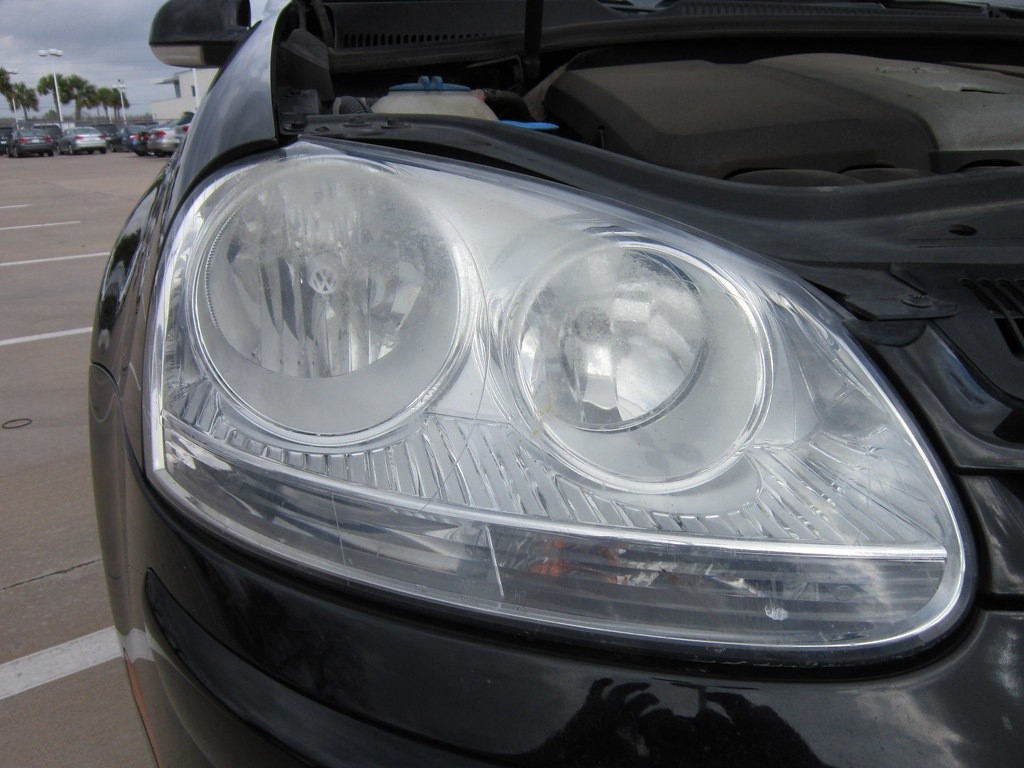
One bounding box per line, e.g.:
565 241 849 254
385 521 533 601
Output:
75 0 1024 767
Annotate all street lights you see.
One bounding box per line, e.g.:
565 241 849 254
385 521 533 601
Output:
111 76 133 127
36 46 67 136
1 64 23 130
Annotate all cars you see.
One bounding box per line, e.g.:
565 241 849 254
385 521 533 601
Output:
0 123 18 156
111 123 149 153
7 128 54 159
56 126 107 155
175 109 197 144
122 118 175 157
